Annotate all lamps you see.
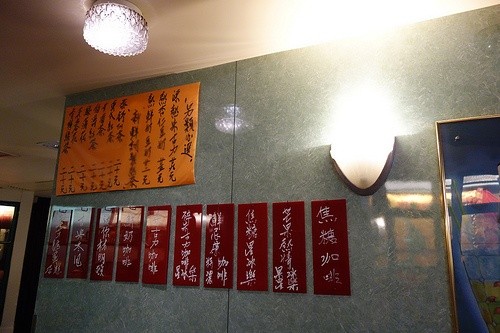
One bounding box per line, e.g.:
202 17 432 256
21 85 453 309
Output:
83 0 148 57
